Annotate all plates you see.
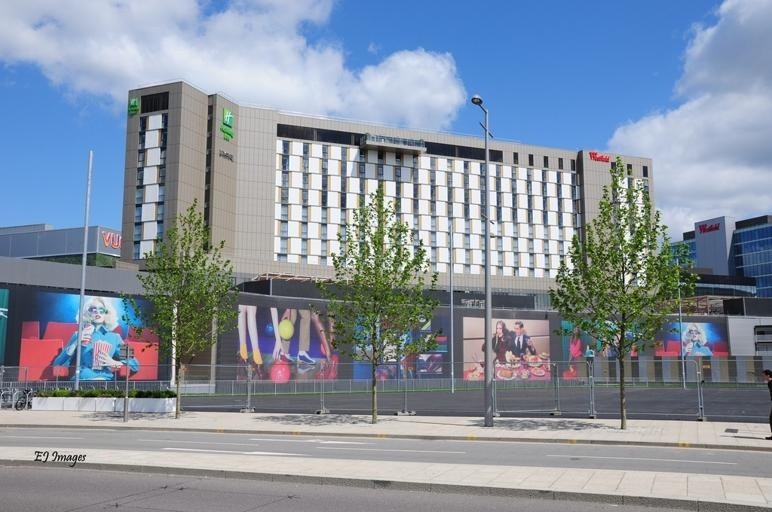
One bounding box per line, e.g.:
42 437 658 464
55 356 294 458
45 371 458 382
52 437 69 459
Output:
496 354 550 381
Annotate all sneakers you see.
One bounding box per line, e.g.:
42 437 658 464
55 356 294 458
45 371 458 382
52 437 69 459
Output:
278 352 294 363
297 352 317 365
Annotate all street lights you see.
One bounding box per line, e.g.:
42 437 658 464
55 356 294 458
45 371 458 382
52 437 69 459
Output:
469 95 494 427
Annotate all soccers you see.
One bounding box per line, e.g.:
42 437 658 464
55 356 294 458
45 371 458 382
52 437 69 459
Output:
278 319 294 340
271 359 291 383
265 322 274 336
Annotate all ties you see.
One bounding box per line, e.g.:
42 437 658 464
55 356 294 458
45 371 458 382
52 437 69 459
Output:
516 337 520 349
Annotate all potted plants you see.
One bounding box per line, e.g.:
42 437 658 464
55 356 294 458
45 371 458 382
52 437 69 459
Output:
32 388 179 412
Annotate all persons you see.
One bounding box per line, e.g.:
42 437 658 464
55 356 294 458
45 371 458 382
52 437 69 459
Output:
509 322 536 360
236 305 340 381
51 296 140 381
629 342 638 357
568 325 583 374
681 322 713 356
482 321 514 364
761 370 772 440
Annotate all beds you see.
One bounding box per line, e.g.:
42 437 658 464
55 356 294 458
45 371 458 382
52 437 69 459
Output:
463 357 551 381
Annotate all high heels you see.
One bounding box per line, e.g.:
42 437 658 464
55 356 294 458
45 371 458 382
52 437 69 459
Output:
235 351 270 380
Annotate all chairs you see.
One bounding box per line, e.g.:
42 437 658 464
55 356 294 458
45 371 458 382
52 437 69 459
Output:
656 341 729 356
20 320 159 380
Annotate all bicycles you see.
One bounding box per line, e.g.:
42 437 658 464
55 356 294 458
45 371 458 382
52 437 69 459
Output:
0 387 38 410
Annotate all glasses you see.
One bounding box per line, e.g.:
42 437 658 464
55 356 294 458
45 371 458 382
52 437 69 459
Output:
88 306 107 315
688 330 700 335
